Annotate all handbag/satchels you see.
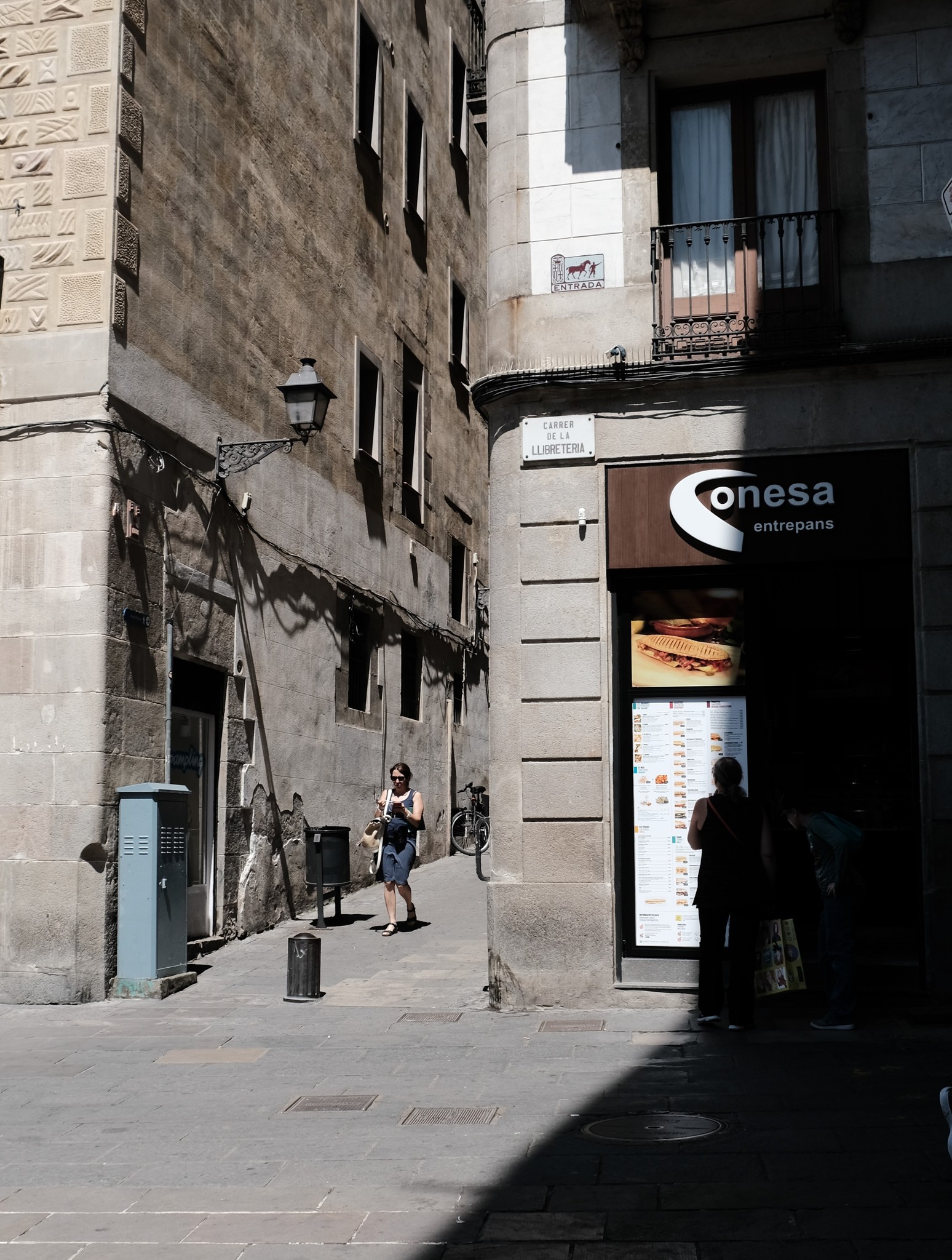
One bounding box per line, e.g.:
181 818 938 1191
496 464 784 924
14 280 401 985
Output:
753 916 807 998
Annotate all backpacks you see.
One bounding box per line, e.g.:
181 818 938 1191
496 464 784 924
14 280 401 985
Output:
808 810 864 849
356 789 393 876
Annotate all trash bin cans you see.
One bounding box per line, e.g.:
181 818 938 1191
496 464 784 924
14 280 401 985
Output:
303 826 351 886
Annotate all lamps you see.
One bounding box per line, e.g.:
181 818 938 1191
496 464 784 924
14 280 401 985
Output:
209 353 341 487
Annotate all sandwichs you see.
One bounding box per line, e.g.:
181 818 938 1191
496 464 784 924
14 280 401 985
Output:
711 733 720 740
645 899 666 903
637 634 732 673
673 721 686 829
676 856 688 905
711 746 721 751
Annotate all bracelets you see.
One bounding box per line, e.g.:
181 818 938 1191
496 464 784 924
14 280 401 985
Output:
377 806 384 813
404 811 410 819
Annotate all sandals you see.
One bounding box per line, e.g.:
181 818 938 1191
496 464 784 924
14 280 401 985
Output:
382 922 398 936
405 903 417 925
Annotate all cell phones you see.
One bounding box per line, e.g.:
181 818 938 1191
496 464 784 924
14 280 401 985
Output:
392 802 402 812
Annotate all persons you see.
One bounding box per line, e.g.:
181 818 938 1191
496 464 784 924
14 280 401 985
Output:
686 757 773 1028
375 762 424 936
785 803 855 1029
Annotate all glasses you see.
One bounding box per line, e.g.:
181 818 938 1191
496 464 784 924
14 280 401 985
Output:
780 810 792 819
390 774 405 781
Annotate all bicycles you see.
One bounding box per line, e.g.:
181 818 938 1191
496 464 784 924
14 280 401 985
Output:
451 781 490 855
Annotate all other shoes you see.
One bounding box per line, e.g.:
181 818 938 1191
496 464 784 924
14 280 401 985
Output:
697 1011 722 1025
808 1012 855 1030
940 1086 952 1160
727 1018 750 1030
